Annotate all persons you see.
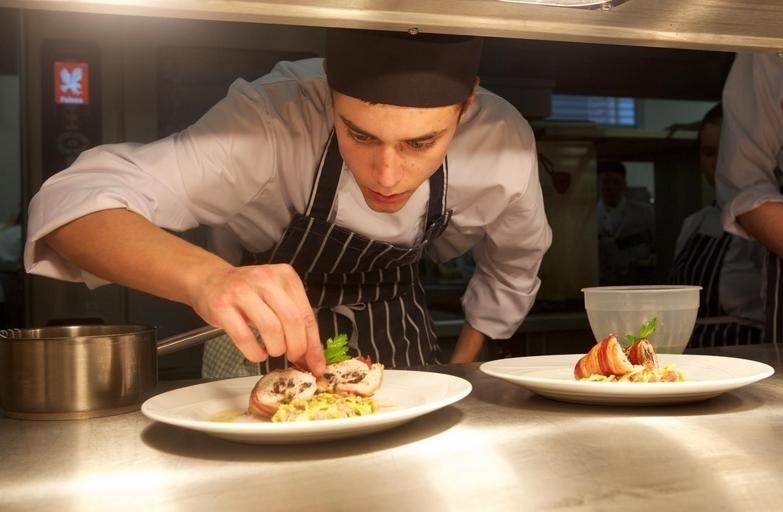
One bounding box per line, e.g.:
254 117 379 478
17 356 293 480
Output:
595 162 655 283
19 29 549 373
665 99 769 350
715 50 783 343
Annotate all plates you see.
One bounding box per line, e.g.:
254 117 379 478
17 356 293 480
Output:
480 353 776 407
141 369 474 444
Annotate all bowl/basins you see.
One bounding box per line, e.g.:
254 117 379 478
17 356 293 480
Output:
581 286 703 353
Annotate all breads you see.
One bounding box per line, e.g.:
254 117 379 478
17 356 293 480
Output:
573 333 658 380
246 358 384 419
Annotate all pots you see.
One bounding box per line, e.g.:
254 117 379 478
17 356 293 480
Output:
0 325 228 421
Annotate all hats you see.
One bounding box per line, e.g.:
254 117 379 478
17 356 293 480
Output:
325 28 484 110
